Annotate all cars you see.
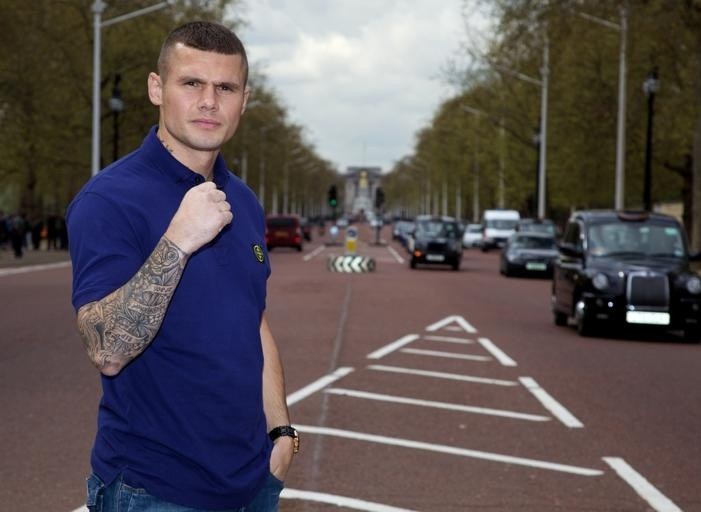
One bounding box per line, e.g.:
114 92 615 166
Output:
391 210 559 278
300 216 313 242
550 208 700 340
266 212 304 252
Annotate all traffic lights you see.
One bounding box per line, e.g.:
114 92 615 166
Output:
327 185 339 208
375 188 385 208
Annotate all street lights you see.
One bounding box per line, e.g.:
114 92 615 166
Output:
641 66 664 212
108 86 124 163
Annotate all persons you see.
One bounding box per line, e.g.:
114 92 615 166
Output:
0 210 70 258
63 20 303 511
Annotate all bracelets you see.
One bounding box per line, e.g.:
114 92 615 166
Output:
269 424 300 456
